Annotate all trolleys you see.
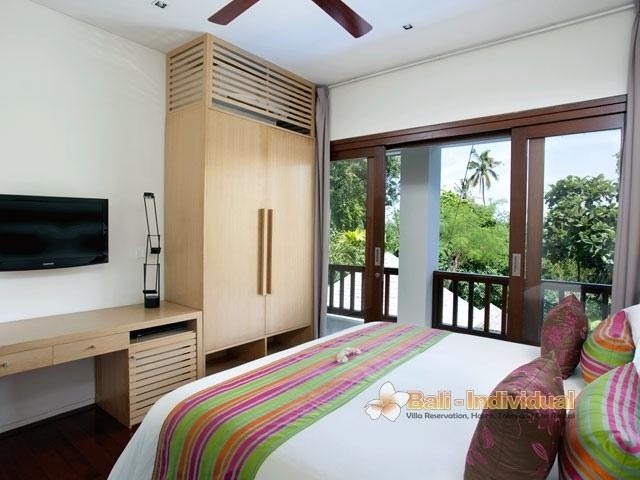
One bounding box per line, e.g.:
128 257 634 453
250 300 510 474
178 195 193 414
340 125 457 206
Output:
208 0 372 39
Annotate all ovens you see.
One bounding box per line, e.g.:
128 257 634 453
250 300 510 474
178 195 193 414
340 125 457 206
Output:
108 319 583 480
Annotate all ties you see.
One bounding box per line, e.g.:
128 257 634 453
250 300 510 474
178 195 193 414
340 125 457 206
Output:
0 193 109 272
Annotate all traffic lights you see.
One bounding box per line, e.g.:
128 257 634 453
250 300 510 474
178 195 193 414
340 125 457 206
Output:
463 293 640 480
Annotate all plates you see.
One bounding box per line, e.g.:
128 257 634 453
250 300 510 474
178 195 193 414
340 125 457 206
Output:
0 300 204 430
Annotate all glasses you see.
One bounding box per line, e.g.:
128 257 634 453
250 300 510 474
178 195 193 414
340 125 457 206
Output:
143 192 161 308
165 33 316 378
